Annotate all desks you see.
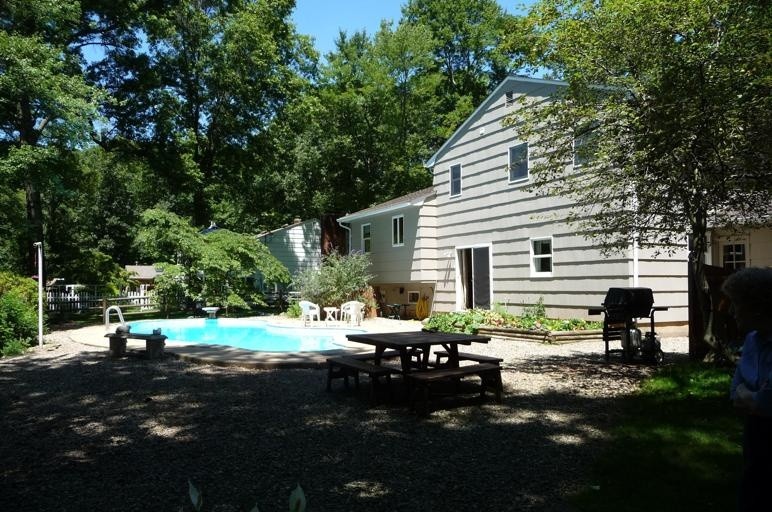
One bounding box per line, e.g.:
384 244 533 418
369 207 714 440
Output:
348 332 490 402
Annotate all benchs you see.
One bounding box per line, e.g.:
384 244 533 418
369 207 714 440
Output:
202 306 221 319
343 349 423 387
104 333 167 360
435 350 502 389
326 358 389 408
408 363 502 411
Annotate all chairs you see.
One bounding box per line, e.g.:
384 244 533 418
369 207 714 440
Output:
299 300 366 327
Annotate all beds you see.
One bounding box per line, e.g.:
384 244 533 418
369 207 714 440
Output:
386 304 411 320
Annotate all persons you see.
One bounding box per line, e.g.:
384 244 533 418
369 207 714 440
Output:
719 266 772 511
